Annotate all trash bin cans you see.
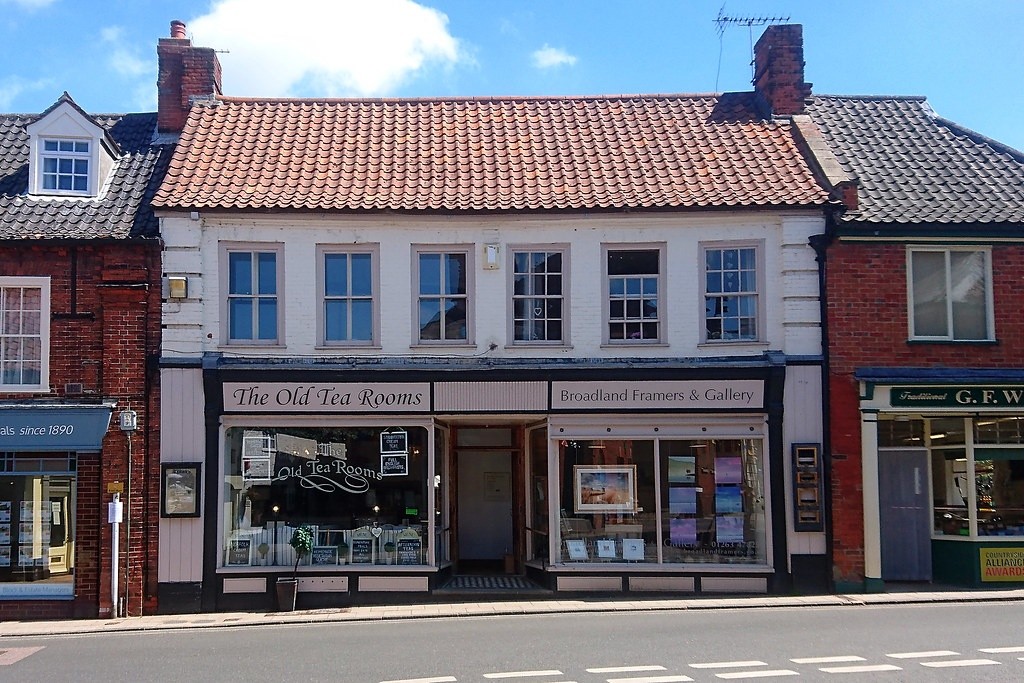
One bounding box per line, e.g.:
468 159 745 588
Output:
505 555 514 574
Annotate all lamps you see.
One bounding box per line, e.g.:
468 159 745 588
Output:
168 276 189 300
688 441 707 448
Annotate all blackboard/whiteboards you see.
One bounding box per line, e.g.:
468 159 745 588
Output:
381 454 408 476
350 528 375 565
396 529 422 565
310 546 338 565
380 431 408 453
226 539 252 567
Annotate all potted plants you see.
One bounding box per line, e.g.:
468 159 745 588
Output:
258 543 269 566
275 526 314 613
384 542 395 566
337 542 348 566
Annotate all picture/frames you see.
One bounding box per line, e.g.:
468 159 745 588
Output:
226 539 253 567
312 546 338 566
350 537 374 564
397 537 423 566
573 463 639 515
